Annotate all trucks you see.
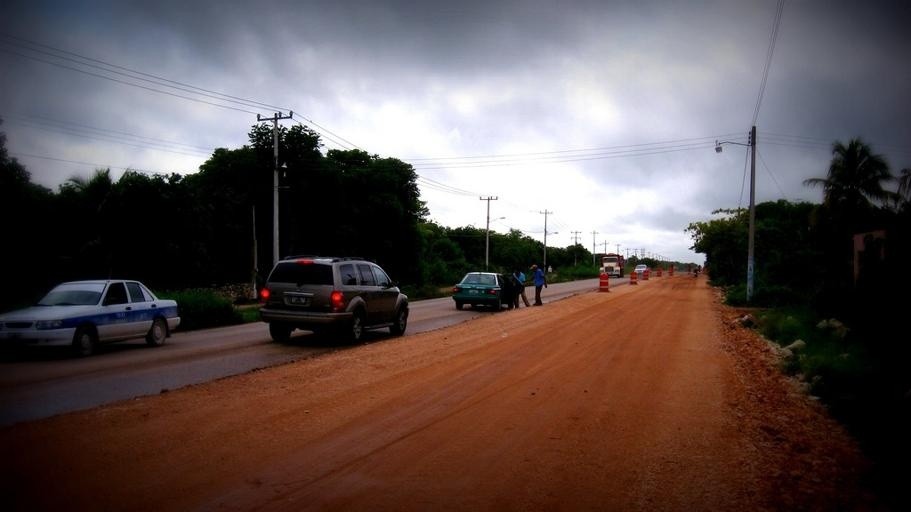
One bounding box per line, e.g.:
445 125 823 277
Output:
601 253 625 280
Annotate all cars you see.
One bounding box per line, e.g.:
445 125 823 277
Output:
450 270 524 311
634 265 648 274
0 279 182 354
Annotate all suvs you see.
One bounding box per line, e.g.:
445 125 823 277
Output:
261 253 410 345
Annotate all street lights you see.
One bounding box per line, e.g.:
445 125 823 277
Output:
714 124 761 300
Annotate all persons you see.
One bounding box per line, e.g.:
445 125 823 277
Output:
531 264 548 304
514 269 530 308
